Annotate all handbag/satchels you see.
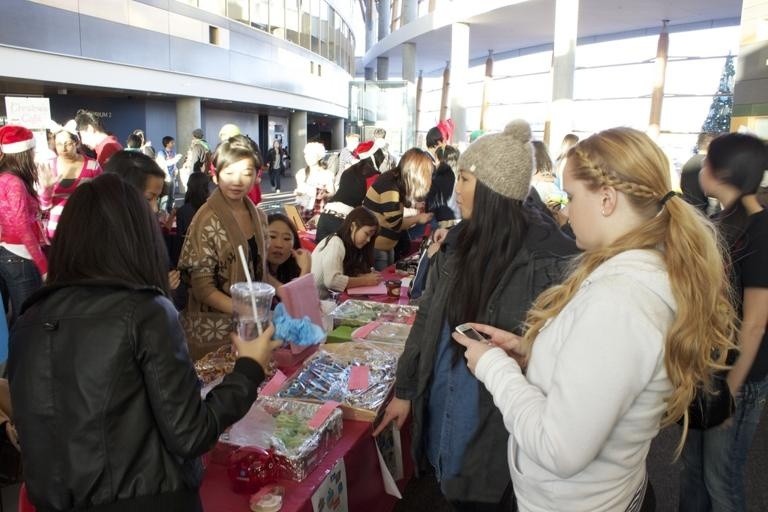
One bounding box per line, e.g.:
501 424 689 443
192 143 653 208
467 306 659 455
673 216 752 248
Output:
674 372 737 430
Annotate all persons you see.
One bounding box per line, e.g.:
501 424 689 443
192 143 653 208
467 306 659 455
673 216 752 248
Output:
2 107 457 512
373 120 768 512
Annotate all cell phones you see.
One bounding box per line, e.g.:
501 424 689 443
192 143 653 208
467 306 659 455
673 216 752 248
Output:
455 323 489 344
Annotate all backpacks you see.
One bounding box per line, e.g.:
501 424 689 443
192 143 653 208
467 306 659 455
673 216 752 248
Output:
406 239 434 305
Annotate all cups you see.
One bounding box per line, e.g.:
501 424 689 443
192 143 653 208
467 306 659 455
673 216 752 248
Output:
229 281 275 342
384 278 401 300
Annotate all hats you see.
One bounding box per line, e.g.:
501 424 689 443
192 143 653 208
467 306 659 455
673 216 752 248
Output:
0 125 35 154
218 123 240 141
351 140 381 161
192 129 203 139
455 120 533 203
304 142 326 157
